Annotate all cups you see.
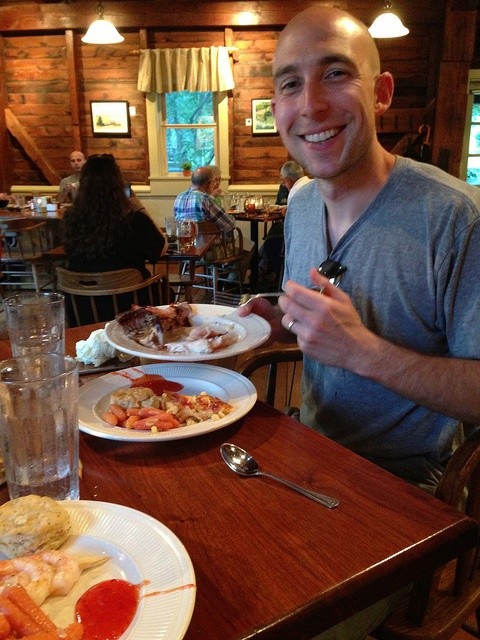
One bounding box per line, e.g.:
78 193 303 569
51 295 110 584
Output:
1 293 66 360
162 216 178 236
15 193 26 211
178 218 198 251
1 353 80 502
230 193 265 212
31 189 40 200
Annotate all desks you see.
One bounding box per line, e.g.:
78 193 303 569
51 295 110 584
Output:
0 202 72 219
235 211 283 276
0 319 480 640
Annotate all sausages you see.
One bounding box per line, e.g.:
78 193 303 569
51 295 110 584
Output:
99 412 118 426
7 584 57 633
125 404 180 429
0 593 45 640
109 403 126 420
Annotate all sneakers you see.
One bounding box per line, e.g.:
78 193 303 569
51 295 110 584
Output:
222 284 249 295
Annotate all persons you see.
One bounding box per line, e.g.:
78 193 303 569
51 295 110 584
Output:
173 166 253 294
54 150 86 204
281 161 314 207
249 160 288 290
208 165 226 214
240 6 480 639
61 152 176 329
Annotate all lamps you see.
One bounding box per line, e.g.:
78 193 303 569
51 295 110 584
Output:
81 0 124 45
369 1 409 40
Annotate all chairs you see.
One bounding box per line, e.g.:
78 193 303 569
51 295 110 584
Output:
237 347 480 640
54 266 171 328
174 220 244 304
0 221 54 304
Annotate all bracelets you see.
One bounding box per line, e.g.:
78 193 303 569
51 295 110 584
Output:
136 206 146 213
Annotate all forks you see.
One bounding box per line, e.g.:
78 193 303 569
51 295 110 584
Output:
211 281 326 308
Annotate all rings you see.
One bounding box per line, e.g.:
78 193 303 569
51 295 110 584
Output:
285 318 297 330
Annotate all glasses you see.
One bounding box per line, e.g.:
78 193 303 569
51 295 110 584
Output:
86 153 116 162
216 178 223 183
309 259 347 294
279 175 289 182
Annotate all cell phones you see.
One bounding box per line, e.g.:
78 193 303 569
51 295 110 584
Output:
123 185 133 199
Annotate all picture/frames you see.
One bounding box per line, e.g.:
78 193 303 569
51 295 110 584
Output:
89 100 131 136
251 97 278 135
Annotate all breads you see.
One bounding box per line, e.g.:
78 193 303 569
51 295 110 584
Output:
111 387 160 409
0 494 71 559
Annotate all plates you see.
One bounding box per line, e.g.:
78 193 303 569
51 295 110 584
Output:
77 364 258 443
60 501 197 639
104 303 272 360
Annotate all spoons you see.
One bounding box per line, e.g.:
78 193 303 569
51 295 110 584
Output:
220 440 341 512
